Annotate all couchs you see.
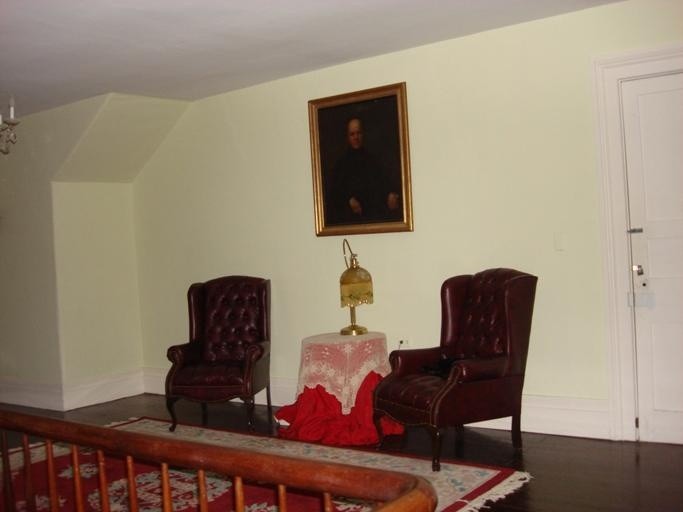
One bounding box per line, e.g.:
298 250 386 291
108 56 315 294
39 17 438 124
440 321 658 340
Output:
162 275 276 435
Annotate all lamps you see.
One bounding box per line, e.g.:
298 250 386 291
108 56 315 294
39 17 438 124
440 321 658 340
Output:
334 239 374 338
0 94 22 156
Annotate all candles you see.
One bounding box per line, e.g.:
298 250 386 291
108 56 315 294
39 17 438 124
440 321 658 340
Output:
8 96 15 119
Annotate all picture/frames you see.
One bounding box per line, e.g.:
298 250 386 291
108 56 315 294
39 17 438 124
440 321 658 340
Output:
307 82 418 237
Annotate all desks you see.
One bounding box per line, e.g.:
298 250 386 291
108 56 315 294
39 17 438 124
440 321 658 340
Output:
270 330 403 447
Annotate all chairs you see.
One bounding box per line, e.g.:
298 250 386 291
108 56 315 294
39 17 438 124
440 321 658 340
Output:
372 266 538 471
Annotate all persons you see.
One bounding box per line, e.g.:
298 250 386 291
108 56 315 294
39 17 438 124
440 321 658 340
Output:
332 114 399 226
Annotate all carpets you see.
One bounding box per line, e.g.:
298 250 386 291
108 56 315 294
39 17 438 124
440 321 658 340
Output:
1 414 534 512
